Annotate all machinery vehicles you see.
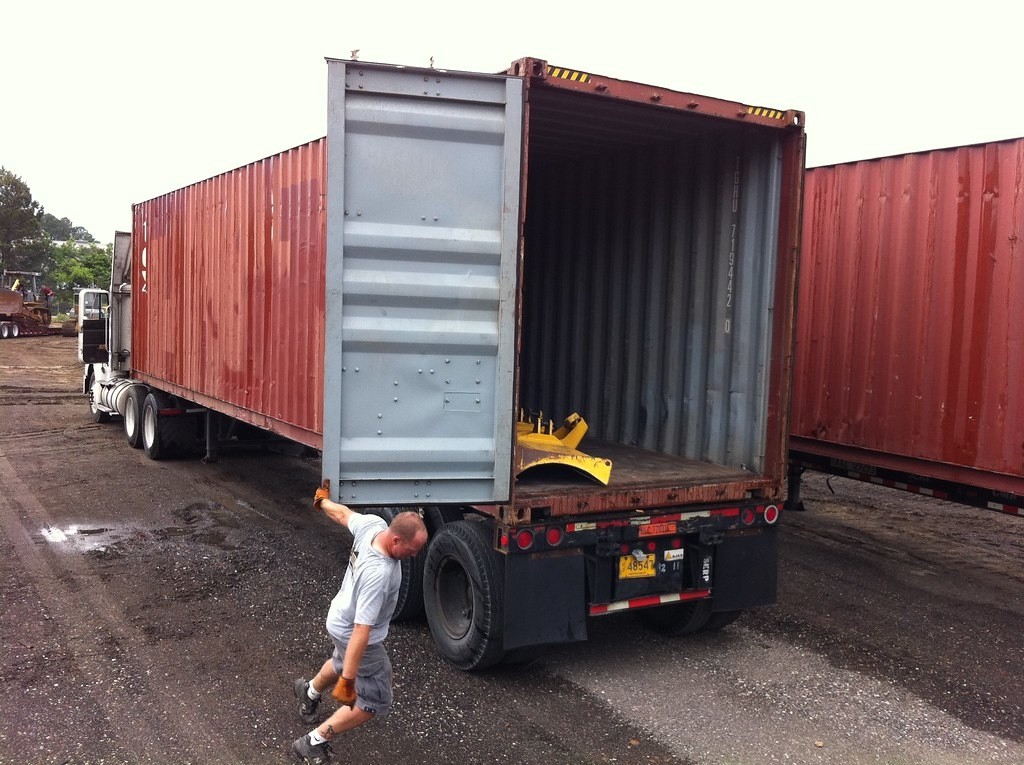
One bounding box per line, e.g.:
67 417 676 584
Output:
61 292 103 337
0 269 52 337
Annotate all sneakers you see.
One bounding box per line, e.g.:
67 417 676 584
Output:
291 734 335 765
293 678 323 724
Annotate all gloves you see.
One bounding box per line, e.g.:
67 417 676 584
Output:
332 675 357 712
313 479 330 513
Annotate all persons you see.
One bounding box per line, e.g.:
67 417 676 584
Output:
41 285 53 309
291 479 427 765
12 279 26 297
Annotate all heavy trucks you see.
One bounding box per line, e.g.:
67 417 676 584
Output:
78 58 805 671
